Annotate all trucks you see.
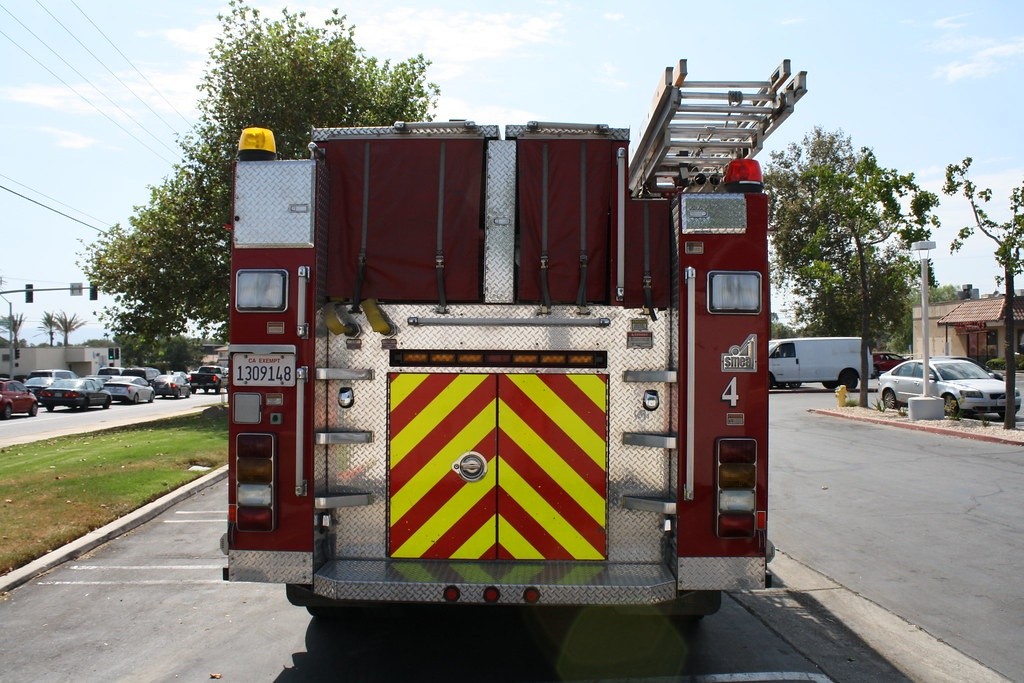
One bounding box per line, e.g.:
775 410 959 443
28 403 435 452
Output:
768 337 873 392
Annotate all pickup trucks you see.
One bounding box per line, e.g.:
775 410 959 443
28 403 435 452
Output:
189 366 229 394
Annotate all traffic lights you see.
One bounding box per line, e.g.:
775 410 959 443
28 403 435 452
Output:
108 348 113 359
115 347 120 359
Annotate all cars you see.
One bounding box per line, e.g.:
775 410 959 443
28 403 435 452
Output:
871 352 910 379
22 367 211 412
0 378 38 420
878 359 1021 418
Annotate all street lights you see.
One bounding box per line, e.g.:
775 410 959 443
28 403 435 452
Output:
908 240 945 421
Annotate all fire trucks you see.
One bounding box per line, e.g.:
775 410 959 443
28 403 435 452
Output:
220 57 808 626
